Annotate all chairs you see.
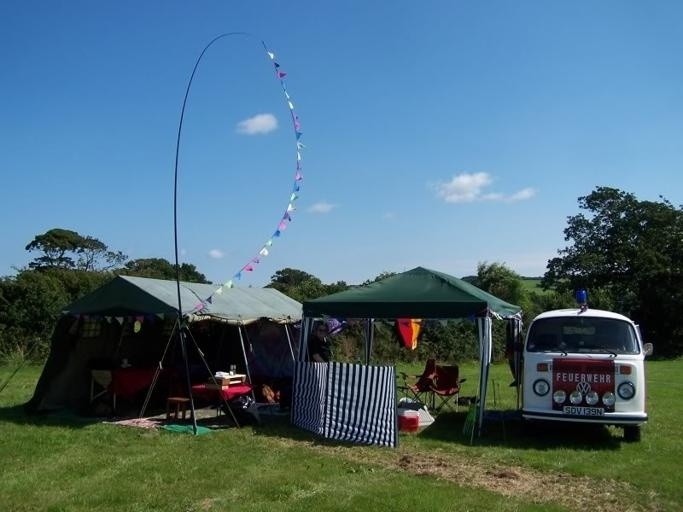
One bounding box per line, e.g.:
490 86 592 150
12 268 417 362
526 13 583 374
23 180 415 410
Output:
407 358 436 408
429 364 460 413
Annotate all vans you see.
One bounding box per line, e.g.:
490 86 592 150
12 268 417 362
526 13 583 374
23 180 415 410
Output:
521 308 654 443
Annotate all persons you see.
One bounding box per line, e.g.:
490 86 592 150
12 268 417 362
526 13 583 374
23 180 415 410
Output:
308 325 331 362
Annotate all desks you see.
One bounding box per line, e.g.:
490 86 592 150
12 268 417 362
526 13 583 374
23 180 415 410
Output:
190 383 252 426
90 368 157 420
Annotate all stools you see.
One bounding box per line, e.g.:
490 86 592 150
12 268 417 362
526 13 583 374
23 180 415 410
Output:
167 397 194 424
